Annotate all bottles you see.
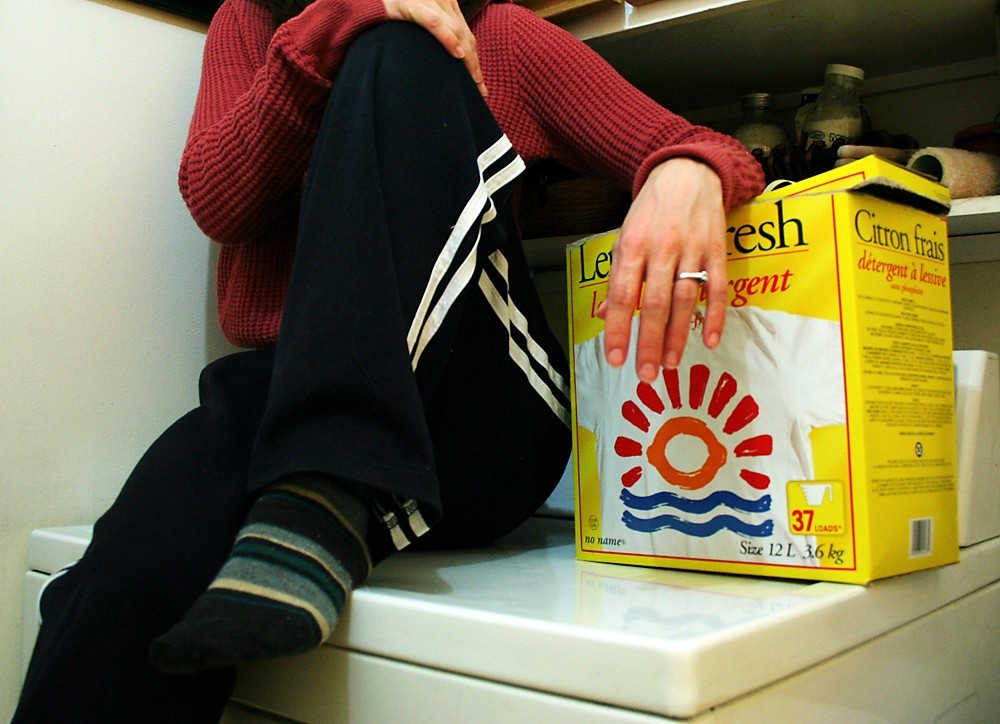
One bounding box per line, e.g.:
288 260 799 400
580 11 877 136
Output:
794 86 822 142
802 64 864 149
732 93 792 158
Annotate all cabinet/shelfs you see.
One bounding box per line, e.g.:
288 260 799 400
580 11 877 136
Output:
522 0 1000 262
26 510 998 724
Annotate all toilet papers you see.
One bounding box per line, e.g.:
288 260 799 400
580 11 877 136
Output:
905 146 1000 199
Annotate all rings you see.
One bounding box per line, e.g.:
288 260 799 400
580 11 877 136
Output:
673 271 708 284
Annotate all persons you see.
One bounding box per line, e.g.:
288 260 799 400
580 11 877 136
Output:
8 0 765 724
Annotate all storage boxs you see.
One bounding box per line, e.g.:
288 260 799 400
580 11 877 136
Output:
567 155 960 584
